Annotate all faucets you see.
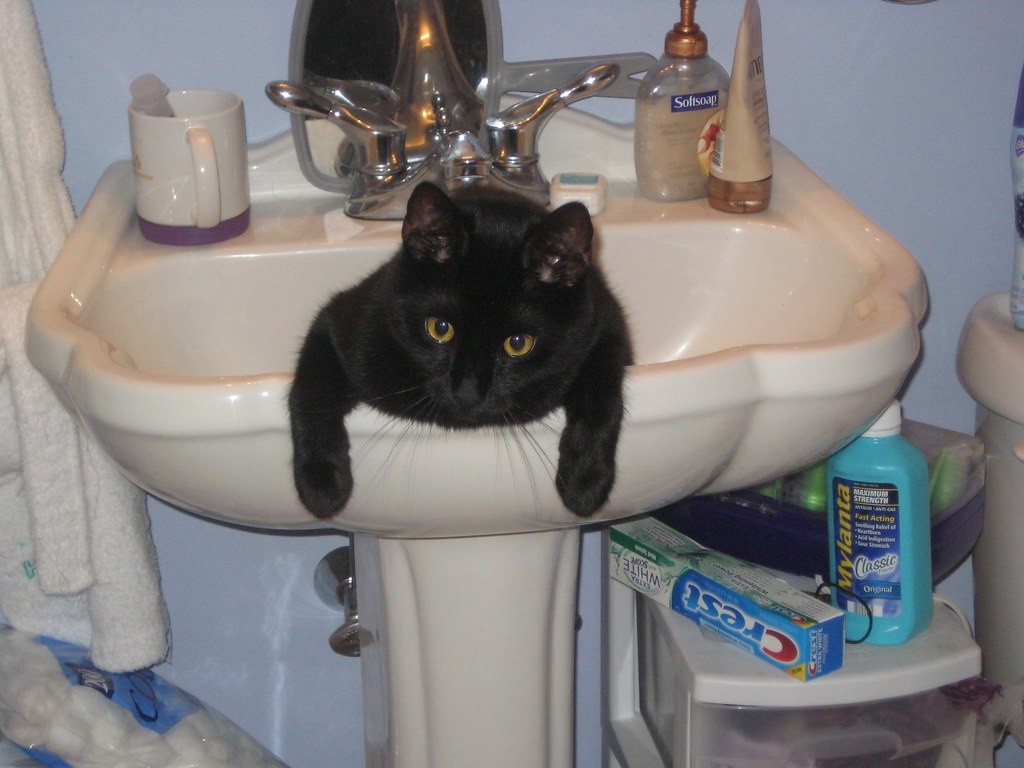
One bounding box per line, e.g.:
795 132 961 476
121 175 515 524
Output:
263 58 619 219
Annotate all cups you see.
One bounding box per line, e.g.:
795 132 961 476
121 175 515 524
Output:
129 89 252 245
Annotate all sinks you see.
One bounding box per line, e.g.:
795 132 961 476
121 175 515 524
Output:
24 184 933 540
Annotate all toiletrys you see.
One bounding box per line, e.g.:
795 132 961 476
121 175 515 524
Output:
633 0 779 216
607 507 847 684
1006 62 1024 331
826 399 934 647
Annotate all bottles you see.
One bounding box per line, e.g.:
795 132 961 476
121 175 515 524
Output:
825 394 933 646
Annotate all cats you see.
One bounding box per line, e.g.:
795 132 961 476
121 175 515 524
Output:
281 180 638 519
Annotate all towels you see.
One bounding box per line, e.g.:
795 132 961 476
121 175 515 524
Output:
0 0 171 681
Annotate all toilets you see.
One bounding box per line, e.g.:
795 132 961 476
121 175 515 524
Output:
938 287 1024 768
